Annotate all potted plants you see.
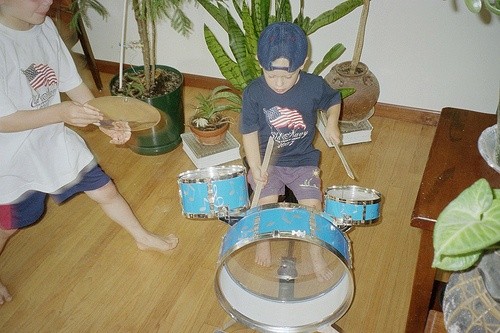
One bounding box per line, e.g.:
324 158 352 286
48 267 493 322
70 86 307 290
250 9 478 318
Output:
431 178 500 333
65 0 230 157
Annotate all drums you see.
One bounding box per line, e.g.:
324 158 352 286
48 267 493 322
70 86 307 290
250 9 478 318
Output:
210 201 357 331
321 184 385 228
177 163 251 222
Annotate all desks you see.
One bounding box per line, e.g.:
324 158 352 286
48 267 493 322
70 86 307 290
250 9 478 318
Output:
404 107 500 333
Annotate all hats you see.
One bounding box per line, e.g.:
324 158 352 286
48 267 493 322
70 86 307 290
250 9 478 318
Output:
257 21 308 73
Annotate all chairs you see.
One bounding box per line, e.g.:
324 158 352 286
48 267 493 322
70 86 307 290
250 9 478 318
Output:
46 0 103 93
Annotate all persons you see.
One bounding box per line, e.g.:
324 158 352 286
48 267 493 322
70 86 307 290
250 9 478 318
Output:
240 22 342 281
0 0 179 306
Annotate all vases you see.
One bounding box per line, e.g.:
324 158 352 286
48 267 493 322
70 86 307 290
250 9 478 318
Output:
190 117 230 144
324 60 380 121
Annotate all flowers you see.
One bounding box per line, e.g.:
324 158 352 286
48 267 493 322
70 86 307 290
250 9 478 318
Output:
187 90 235 129
